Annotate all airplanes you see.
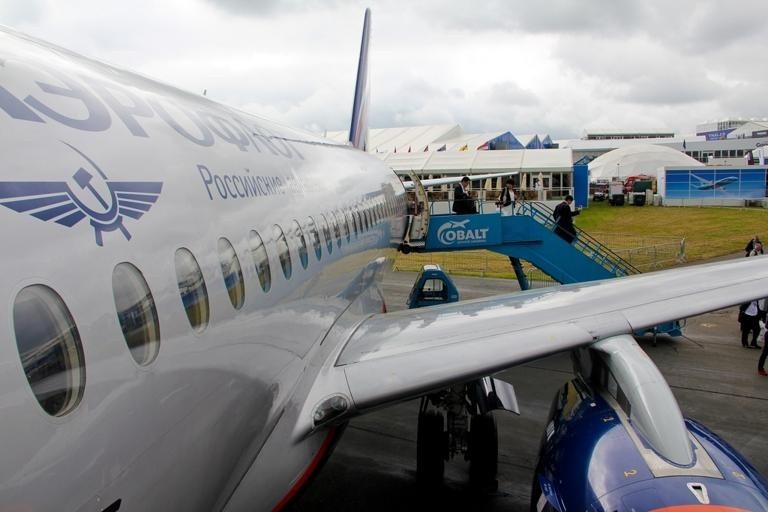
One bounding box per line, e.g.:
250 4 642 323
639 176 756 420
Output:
685 172 739 195
0 5 768 512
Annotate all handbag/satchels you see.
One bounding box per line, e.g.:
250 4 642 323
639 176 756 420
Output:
496 195 506 203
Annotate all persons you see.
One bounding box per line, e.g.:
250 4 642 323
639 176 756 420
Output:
552 195 582 244
749 243 762 257
745 235 763 257
452 177 470 214
758 321 768 376
468 191 480 214
738 300 761 349
758 297 768 323
500 179 518 216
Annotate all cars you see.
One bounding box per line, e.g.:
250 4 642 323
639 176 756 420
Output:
592 174 656 201
